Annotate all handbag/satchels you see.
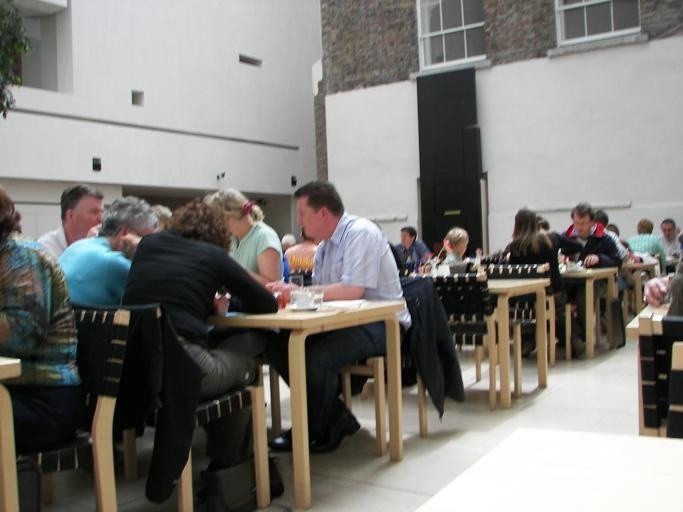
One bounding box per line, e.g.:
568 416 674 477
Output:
215 458 283 512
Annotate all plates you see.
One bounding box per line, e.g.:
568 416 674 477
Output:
289 304 319 312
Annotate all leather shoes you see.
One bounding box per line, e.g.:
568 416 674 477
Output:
270 412 360 452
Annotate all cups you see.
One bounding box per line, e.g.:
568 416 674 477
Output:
303 288 324 308
290 291 315 306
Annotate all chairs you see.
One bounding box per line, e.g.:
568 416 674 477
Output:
630 314 683 443
121 302 277 507
18 308 131 512
421 274 498 410
488 263 575 367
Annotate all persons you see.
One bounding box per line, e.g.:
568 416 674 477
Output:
56 195 160 309
503 202 683 348
264 180 412 455
36 183 105 264
152 204 318 282
374 220 468 272
1 192 83 457
120 198 278 512
